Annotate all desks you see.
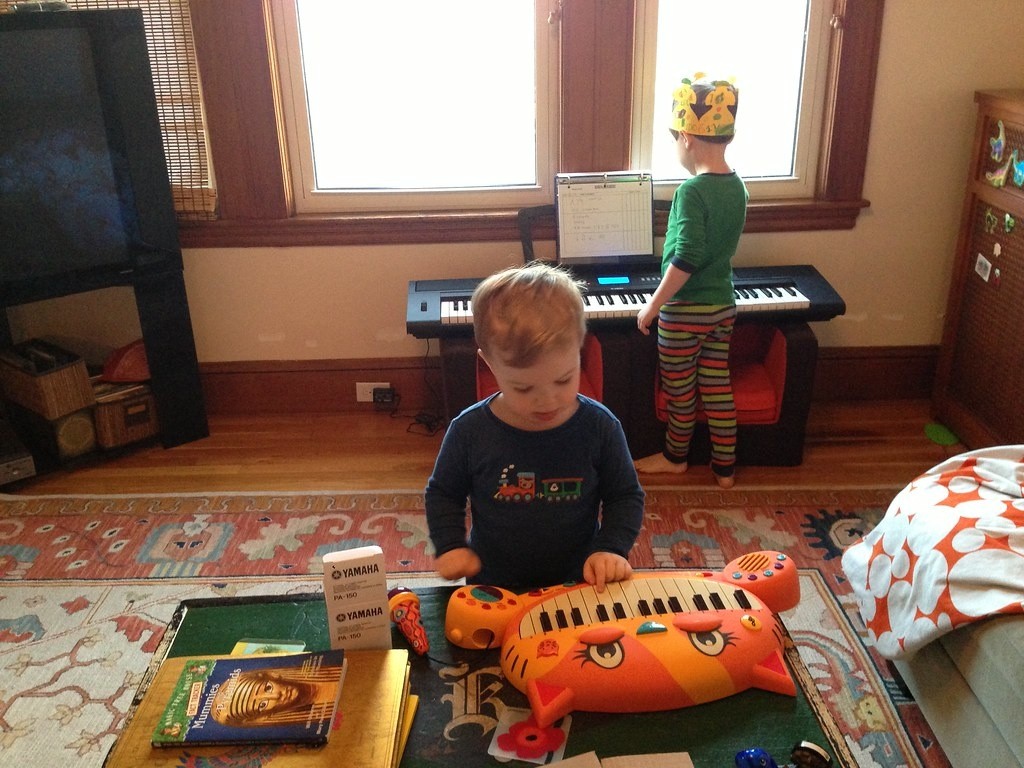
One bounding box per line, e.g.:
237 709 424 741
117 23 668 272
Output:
100 584 864 768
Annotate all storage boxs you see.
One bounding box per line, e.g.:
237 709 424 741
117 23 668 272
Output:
0 338 158 482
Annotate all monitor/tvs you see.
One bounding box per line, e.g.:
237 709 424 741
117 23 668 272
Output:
0 9 184 303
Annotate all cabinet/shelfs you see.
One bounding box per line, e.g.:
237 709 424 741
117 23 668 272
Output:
931 92 1024 455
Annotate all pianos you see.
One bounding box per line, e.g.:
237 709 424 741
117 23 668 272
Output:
444 546 811 730
401 261 849 342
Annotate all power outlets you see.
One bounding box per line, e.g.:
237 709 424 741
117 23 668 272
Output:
356 382 390 402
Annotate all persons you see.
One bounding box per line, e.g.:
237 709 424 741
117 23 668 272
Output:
423 260 646 592
632 82 751 489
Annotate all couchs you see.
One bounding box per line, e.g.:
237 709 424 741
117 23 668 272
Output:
628 317 819 468
439 328 628 455
890 612 1024 768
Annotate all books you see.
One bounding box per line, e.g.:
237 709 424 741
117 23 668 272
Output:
104 648 419 768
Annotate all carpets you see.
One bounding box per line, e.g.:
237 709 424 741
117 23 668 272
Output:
0 481 954 768
